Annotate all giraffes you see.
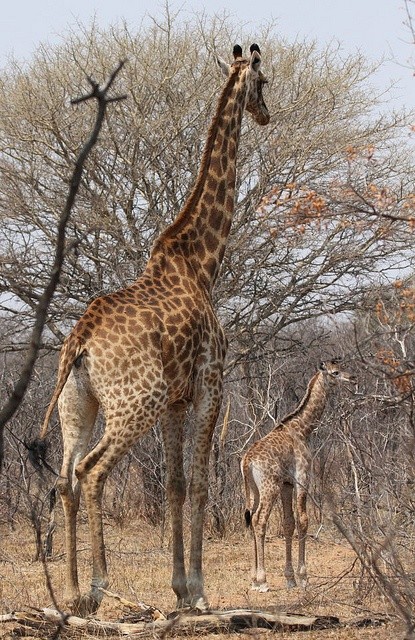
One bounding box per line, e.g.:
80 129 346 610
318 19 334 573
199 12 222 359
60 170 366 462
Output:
26 44 271 615
240 360 359 594
